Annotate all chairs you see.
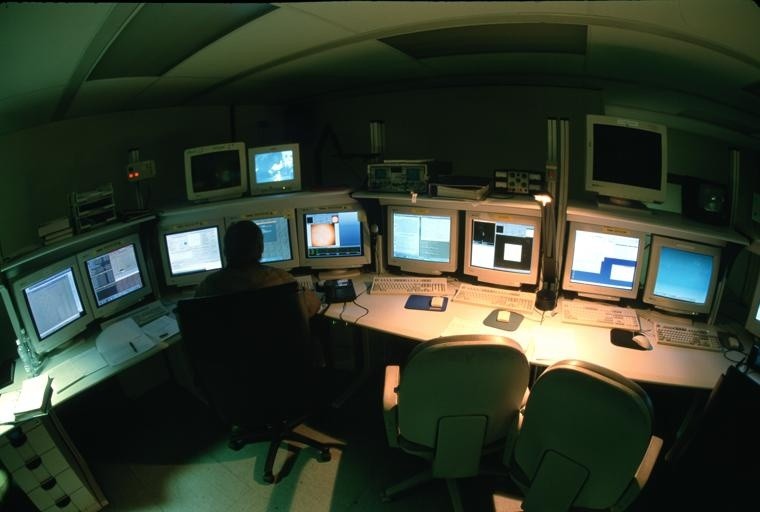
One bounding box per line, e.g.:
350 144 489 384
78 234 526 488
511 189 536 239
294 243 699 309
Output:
378 333 531 511
172 280 329 487
663 363 760 489
506 357 664 512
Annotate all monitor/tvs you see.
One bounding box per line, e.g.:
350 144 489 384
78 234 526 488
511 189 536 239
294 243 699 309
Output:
296 204 372 281
11 255 95 355
183 142 249 203
387 205 460 276
463 209 543 285
643 236 721 315
156 216 225 288
0 284 22 366
247 142 302 197
745 280 760 338
76 232 153 319
562 207 645 301
225 207 300 270
585 112 668 211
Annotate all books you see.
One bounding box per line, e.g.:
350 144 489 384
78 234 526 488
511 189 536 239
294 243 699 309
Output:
140 314 180 344
94 318 155 368
45 347 108 395
0 374 54 425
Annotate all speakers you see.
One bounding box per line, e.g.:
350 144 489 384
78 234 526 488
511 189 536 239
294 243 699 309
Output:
679 181 731 228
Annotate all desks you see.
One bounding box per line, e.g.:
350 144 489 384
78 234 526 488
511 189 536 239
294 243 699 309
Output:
312 267 754 468
9 287 182 409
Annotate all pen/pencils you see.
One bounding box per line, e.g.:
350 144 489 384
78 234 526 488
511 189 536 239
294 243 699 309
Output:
128 341 138 354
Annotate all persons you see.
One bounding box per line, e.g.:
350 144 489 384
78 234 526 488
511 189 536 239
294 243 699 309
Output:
193 220 321 323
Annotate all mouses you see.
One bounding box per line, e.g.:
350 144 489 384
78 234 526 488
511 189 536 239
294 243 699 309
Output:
496 310 510 322
430 296 444 308
632 335 653 350
729 336 739 349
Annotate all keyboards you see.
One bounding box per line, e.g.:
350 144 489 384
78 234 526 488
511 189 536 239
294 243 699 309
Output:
452 284 536 313
0 359 16 388
370 277 447 296
557 298 641 331
98 299 171 331
654 322 723 352
293 275 314 291
